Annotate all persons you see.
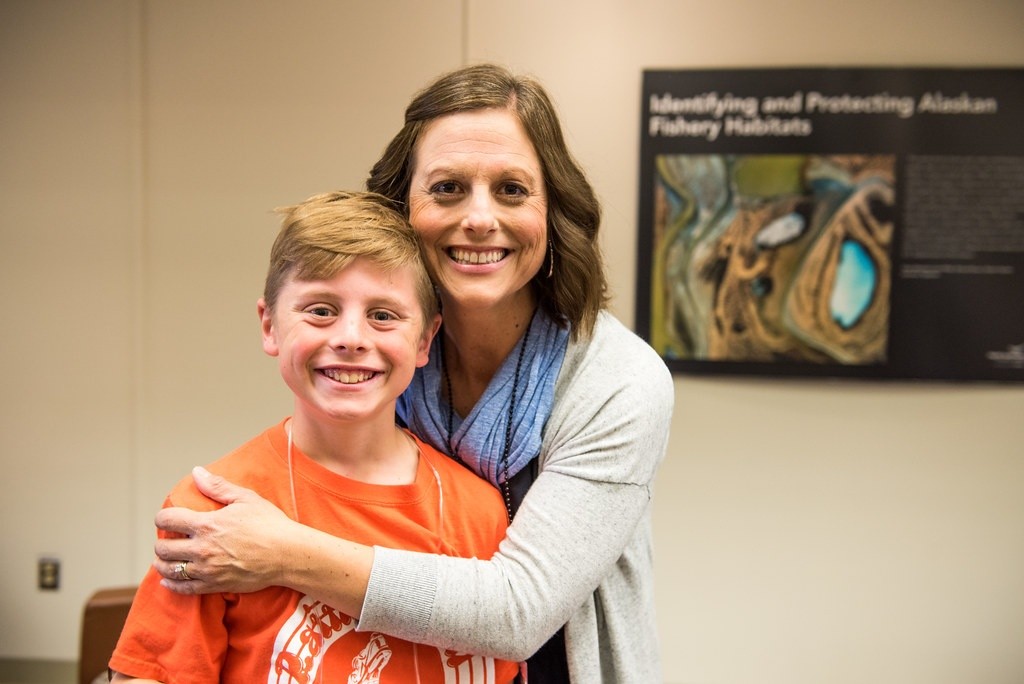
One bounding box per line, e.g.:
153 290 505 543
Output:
148 65 679 684
105 193 530 684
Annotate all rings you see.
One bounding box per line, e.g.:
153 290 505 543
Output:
179 562 190 580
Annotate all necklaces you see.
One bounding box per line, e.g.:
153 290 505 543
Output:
442 308 539 523
287 422 444 683
